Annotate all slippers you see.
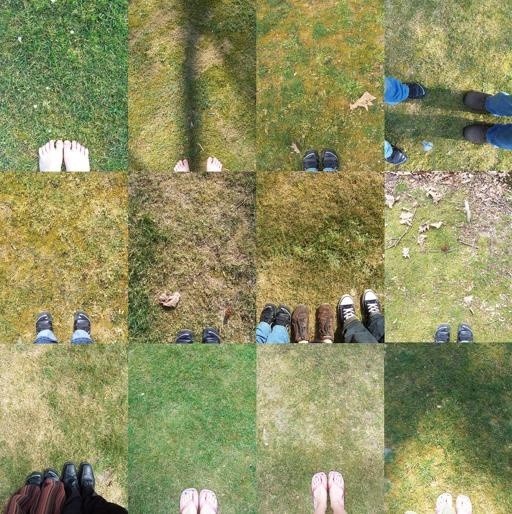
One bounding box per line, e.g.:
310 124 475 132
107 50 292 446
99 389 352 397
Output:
328 470 345 510
311 471 328 512
436 492 453 514
179 487 199 514
455 494 473 514
199 488 218 514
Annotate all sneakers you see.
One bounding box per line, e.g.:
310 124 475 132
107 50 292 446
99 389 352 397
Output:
301 150 320 169
274 304 291 332
403 82 426 100
385 144 407 165
35 311 54 338
361 288 382 324
44 469 60 484
462 91 494 113
337 292 359 328
462 123 495 146
73 311 91 339
455 322 474 343
174 329 194 344
433 323 452 342
259 302 277 327
291 304 311 343
202 327 221 343
317 302 335 342
25 472 42 488
322 149 339 169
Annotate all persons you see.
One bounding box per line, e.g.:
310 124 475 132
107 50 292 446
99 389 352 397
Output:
291 303 336 344
310 470 348 514
461 89 512 153
33 310 97 343
172 156 223 173
434 492 474 514
257 302 291 346
179 486 219 513
385 75 426 166
36 138 91 171
338 290 385 343
6 462 130 514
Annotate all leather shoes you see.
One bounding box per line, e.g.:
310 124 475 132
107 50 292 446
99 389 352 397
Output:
80 463 95 490
62 463 78 488
